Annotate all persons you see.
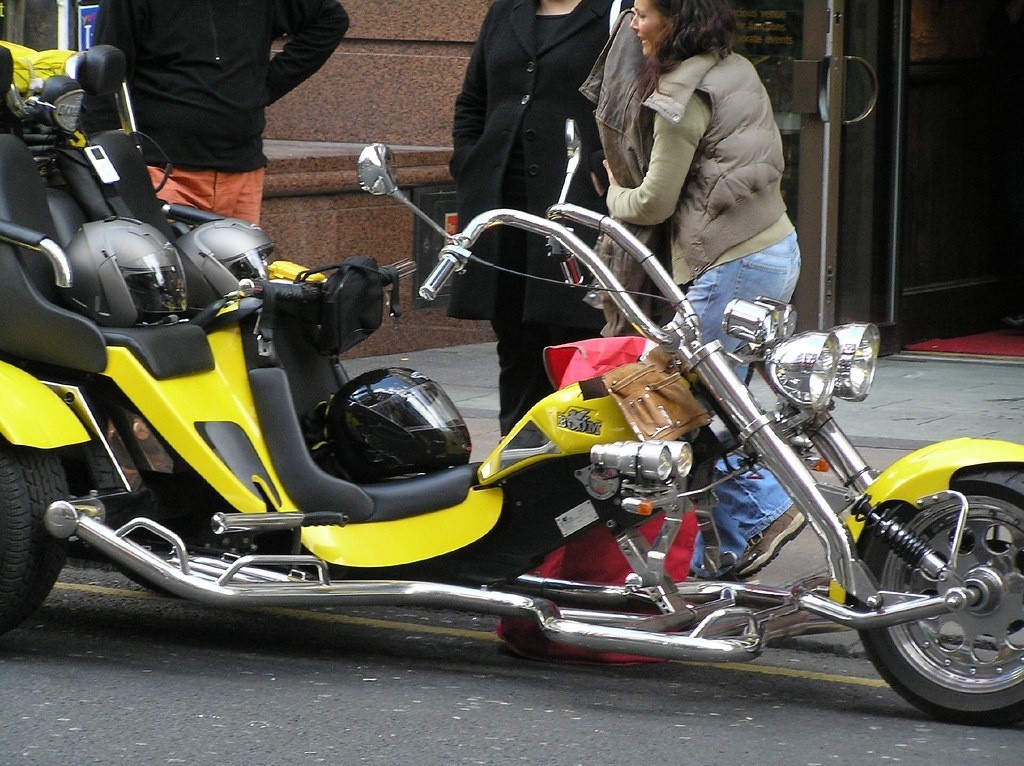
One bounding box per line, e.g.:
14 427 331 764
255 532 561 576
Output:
448 0 808 577
91 0 349 226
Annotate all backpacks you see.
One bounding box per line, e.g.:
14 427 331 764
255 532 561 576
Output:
495 336 699 665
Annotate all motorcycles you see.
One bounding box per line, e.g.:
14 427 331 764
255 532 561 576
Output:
0 40 1024 726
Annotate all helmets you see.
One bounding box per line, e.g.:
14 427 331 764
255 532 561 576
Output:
173 218 283 316
312 367 472 483
48 217 187 329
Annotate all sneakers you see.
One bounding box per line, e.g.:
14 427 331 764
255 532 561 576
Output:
732 503 809 579
